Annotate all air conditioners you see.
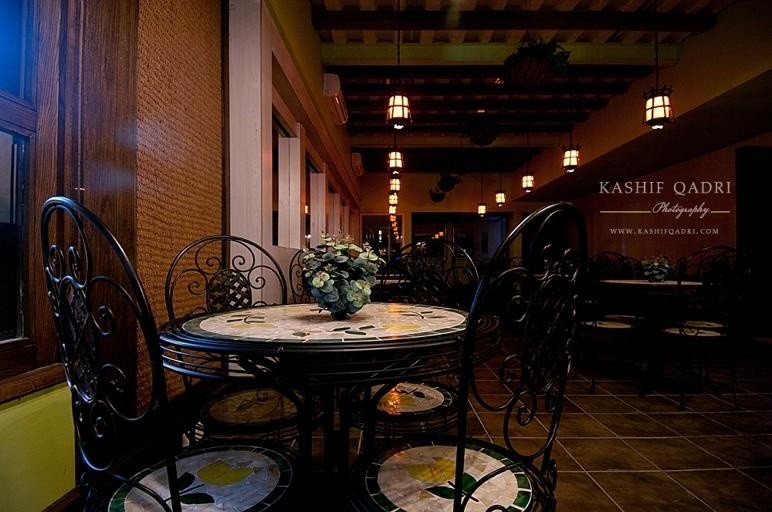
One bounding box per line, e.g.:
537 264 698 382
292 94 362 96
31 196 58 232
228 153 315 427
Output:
321 70 349 127
351 150 366 180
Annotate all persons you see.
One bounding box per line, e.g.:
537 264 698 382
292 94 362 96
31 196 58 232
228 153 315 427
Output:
389 242 402 274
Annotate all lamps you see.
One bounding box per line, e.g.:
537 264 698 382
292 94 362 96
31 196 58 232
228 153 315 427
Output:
386 129 535 241
561 85 582 172
642 1 675 131
387 0 413 131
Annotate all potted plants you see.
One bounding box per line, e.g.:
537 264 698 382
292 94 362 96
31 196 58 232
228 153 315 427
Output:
300 231 388 322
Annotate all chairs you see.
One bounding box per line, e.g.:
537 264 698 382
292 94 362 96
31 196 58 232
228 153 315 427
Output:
166 234 310 447
337 236 479 455
336 200 588 512
36 193 312 510
466 242 757 412
